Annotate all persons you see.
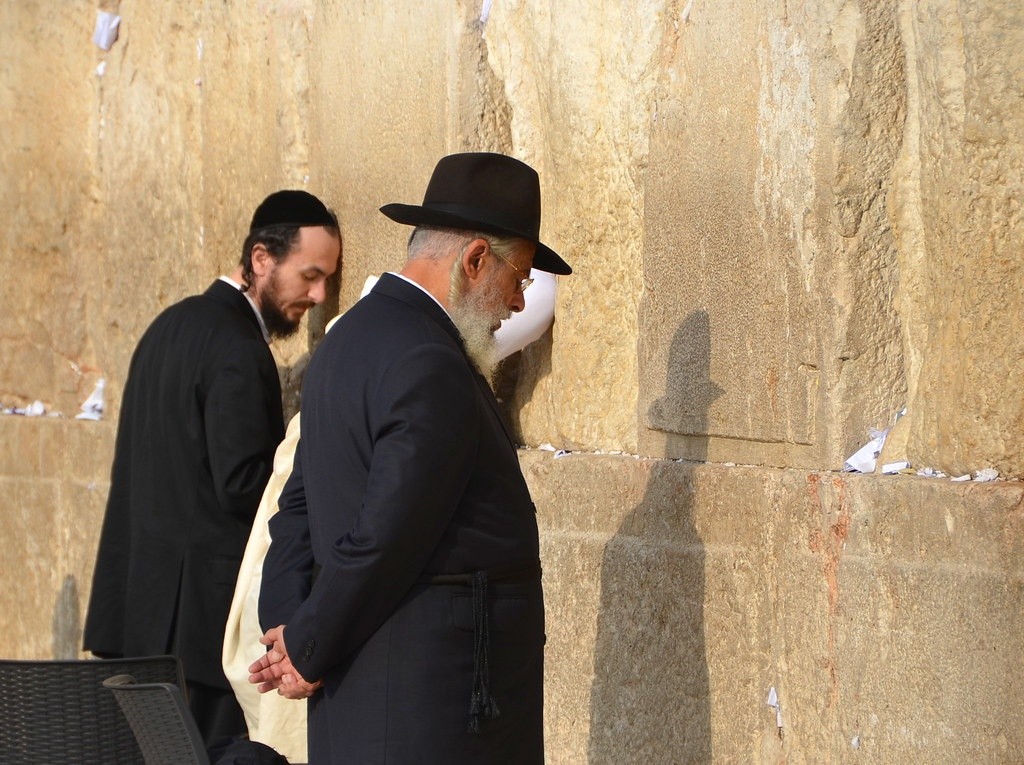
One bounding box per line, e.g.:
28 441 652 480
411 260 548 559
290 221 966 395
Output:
83 187 341 765
248 151 573 765
220 263 556 765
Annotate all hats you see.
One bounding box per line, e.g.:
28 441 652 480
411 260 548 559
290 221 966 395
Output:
379 153 572 275
250 190 336 229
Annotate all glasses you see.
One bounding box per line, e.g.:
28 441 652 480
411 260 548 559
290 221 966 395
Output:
491 246 534 294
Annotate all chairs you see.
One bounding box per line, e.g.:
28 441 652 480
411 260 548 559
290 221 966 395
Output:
0 654 210 765
